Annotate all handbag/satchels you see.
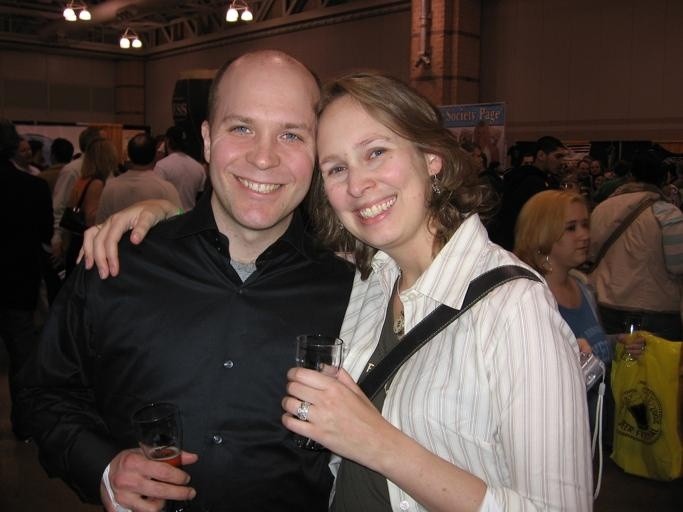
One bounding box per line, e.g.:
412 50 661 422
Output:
60 206 88 235
609 331 683 482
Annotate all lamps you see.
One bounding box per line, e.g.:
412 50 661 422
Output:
119 26 144 49
226 0 254 23
63 0 92 22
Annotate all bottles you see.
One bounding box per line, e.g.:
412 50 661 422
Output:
610 316 644 430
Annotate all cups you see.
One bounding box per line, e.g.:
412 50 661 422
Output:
130 401 197 512
295 335 344 455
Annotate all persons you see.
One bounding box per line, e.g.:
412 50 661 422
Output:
19 47 360 511
1 115 680 511
74 70 599 512
